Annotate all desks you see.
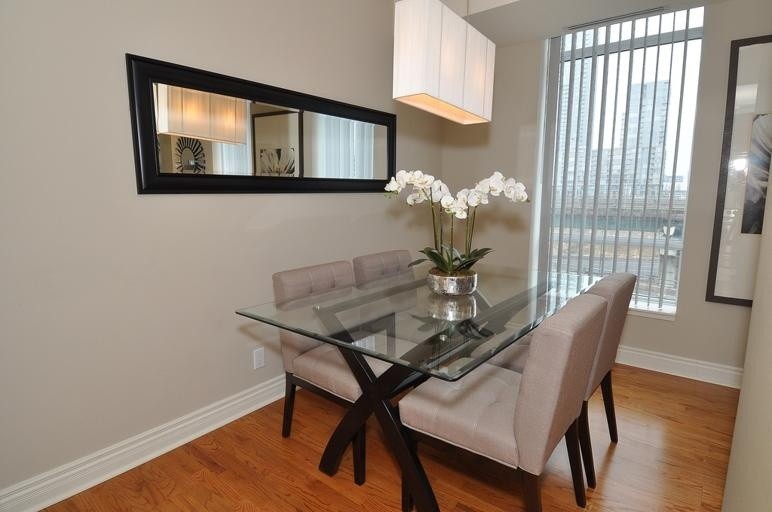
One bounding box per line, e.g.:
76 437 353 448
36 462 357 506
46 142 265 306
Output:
235 267 605 512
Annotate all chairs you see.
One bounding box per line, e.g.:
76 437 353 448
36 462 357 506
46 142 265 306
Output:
271 258 430 487
469 271 635 490
350 250 498 359
391 292 612 510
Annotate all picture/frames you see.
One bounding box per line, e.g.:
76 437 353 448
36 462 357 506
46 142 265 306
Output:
705 35 772 307
251 110 304 176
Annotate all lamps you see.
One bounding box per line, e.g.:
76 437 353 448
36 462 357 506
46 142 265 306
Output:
153 83 247 145
392 0 496 125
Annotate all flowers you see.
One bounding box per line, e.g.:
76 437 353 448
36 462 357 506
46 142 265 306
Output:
384 170 530 275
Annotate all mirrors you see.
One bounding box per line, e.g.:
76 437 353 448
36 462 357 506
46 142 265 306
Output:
125 53 396 195
175 137 205 174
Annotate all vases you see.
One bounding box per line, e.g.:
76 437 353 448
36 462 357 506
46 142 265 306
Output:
425 268 477 296
